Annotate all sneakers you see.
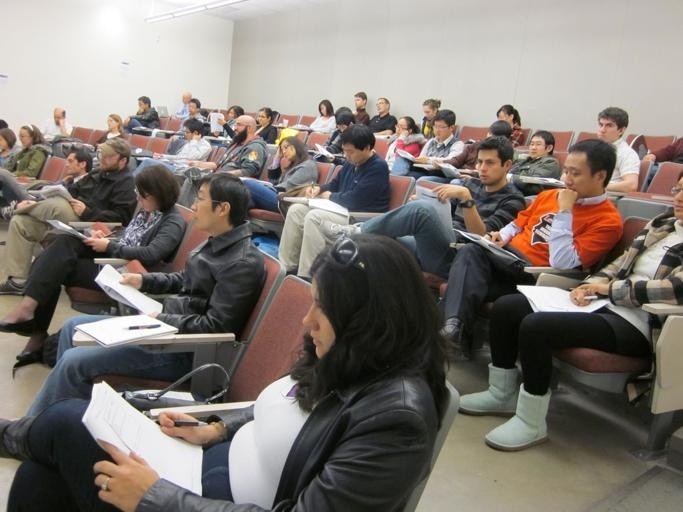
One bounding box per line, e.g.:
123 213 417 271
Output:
439 318 466 355
323 222 357 241
0 277 28 295
184 167 213 184
1 201 16 221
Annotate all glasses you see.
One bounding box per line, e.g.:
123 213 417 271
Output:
328 234 365 271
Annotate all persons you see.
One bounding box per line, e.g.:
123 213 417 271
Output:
0 233 450 510
0 85 683 451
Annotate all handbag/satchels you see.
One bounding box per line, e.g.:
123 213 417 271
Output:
13 330 60 367
277 182 320 213
123 363 229 411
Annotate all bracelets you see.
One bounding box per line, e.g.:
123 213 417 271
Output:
210 419 230 440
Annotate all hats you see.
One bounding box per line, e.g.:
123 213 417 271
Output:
97 138 130 157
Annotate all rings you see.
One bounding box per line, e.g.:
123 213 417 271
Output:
102 476 109 491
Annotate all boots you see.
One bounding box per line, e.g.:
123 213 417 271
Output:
459 364 520 417
484 383 550 451
0 399 68 462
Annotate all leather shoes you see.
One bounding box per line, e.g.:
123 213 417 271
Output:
16 346 42 359
0 318 34 334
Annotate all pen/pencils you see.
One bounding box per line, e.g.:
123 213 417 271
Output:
155 420 200 426
105 230 118 238
311 180 314 198
584 295 598 300
486 232 500 242
128 324 161 330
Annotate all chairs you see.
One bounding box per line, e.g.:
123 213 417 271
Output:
452 124 491 146
383 174 464 296
96 248 460 512
525 214 682 470
248 156 344 238
69 125 228 170
513 127 683 219
12 156 67 249
64 203 212 317
374 137 391 160
158 111 334 151
